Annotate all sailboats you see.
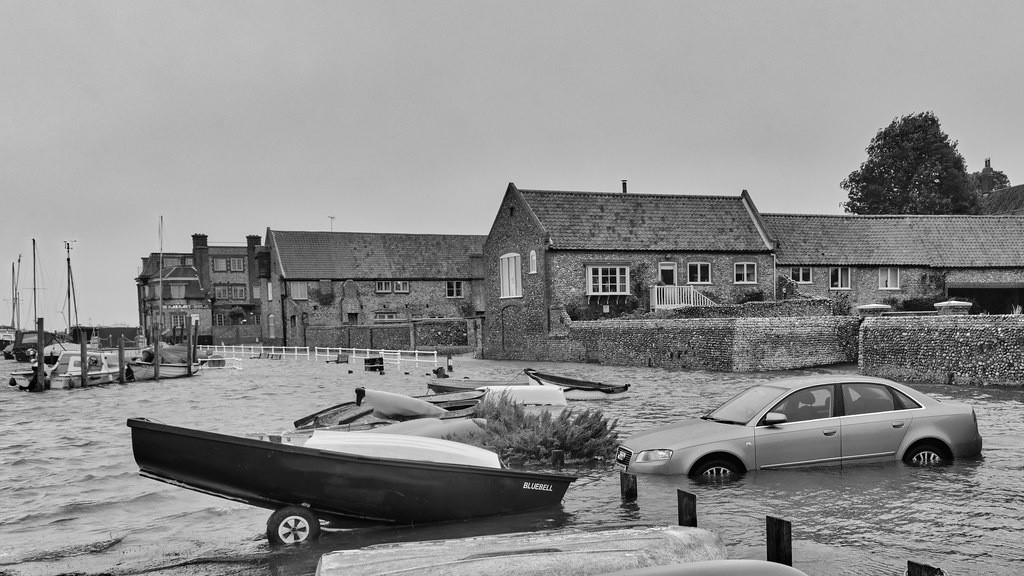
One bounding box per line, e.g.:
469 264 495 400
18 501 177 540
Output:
0 214 203 390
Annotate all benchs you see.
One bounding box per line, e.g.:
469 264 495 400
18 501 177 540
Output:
271 355 281 359
326 353 348 364
854 397 894 412
250 352 269 358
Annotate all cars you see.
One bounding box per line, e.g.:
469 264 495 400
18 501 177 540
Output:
614 374 982 484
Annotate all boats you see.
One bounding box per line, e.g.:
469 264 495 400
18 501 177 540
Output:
523 368 631 401
121 415 579 528
261 378 569 448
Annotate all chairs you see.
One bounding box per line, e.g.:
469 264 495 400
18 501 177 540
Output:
825 397 847 417
795 392 816 418
